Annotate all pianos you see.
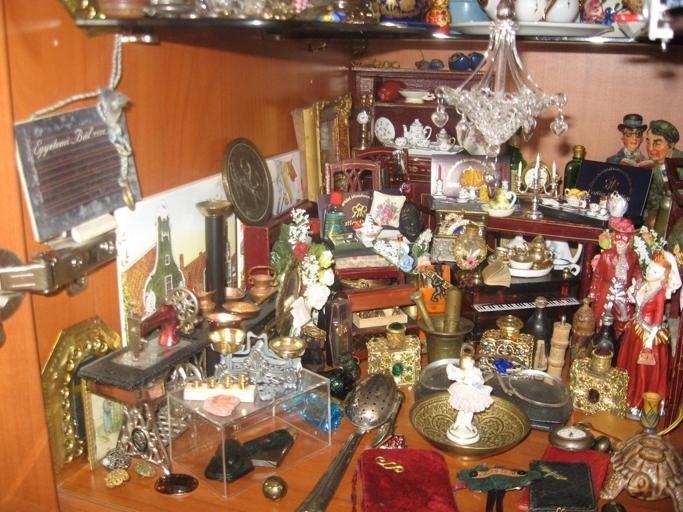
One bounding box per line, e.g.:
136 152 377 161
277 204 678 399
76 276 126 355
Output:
456 271 582 355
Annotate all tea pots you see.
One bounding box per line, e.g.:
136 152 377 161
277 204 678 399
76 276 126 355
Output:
434 128 456 152
522 235 553 270
402 117 432 148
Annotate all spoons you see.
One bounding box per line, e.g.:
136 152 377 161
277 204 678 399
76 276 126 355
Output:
292 371 399 512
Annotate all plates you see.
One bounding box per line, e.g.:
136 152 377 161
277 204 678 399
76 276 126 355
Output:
507 262 554 279
479 203 516 217
445 19 615 39
520 161 552 194
406 390 532 462
373 116 396 146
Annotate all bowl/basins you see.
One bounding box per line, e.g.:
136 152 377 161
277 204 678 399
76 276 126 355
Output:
204 311 242 331
508 254 534 271
221 285 246 301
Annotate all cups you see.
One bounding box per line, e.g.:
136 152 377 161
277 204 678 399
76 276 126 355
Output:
415 138 430 149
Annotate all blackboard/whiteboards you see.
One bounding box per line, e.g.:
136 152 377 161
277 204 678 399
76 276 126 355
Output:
13 104 142 243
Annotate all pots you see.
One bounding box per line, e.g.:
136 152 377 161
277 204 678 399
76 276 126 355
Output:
221 289 278 320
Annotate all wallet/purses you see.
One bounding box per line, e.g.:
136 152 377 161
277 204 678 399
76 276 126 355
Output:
352 448 456 512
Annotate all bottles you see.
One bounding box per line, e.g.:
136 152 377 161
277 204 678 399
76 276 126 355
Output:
590 313 618 370
321 189 346 241
524 296 552 359
561 145 584 197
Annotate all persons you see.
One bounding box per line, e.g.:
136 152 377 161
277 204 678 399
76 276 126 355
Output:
588 215 643 353
607 111 647 169
617 248 682 421
643 120 683 253
